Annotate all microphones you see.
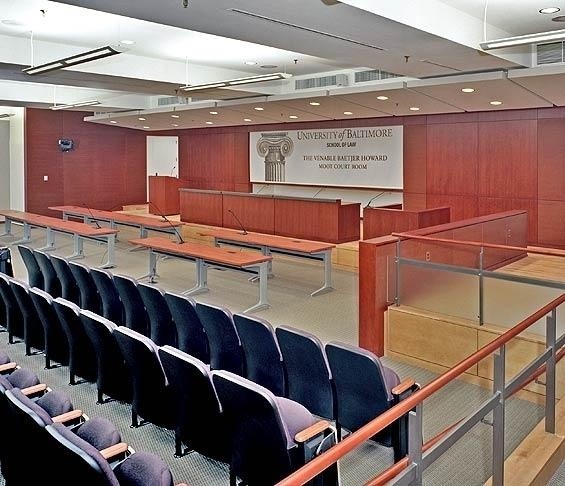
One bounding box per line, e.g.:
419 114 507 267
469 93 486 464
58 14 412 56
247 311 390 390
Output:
81 201 102 230
161 214 184 244
228 209 249 236
311 186 325 199
362 189 385 209
171 165 175 176
255 181 270 195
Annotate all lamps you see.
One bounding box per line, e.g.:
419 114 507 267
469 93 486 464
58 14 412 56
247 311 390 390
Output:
19 31 123 113
180 55 289 92
480 1 565 52
